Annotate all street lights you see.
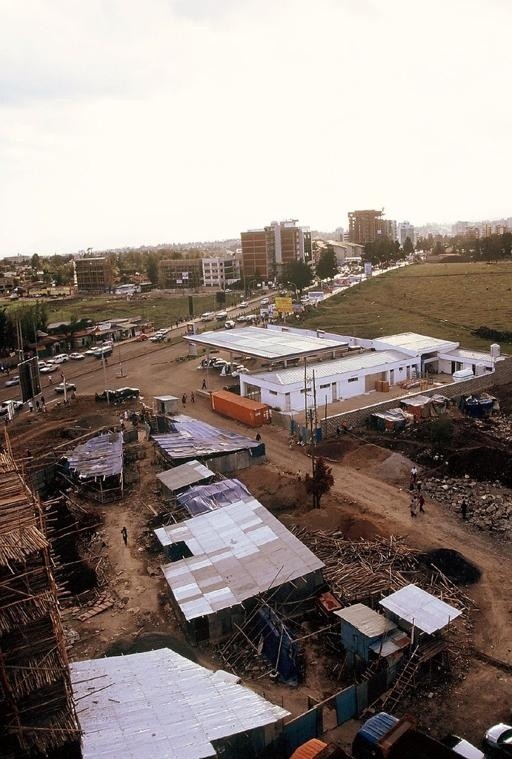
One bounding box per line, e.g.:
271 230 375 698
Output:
287 281 299 300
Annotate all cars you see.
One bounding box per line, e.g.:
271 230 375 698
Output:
200 356 250 378
236 314 258 323
1 400 23 410
239 302 248 308
150 329 167 343
483 723 512 759
70 353 84 360
38 359 60 374
55 382 77 393
5 376 21 387
224 321 235 328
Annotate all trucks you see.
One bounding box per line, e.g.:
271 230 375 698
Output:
216 312 227 321
201 312 215 321
288 711 466 759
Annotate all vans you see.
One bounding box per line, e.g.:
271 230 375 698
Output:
260 298 269 304
440 733 487 759
53 354 69 363
86 341 113 358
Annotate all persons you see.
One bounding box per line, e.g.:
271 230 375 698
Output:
251 299 316 326
183 392 187 400
460 500 468 521
190 391 195 403
256 433 262 441
201 379 206 389
120 526 129 546
407 466 426 518
181 395 187 408
2 369 76 427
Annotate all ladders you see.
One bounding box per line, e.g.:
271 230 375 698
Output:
382 645 425 713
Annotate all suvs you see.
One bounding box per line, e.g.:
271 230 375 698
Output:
115 387 140 398
